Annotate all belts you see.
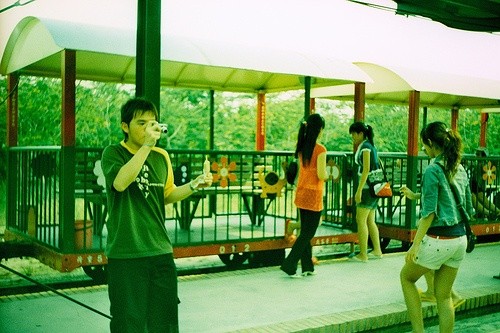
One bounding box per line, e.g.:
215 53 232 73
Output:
427 234 458 240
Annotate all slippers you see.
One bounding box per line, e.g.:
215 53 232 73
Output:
349 256 367 262
419 293 438 301
367 253 384 259
454 298 466 308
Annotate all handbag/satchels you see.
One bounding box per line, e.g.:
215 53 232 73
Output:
467 231 477 253
370 181 394 198
287 155 298 185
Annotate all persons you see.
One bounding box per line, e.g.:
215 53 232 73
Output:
102 97 213 333
349 121 386 263
279 114 329 279
397 121 471 333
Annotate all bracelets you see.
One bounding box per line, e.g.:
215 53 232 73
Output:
190 184 198 191
143 143 153 147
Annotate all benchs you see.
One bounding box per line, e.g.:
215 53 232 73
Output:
377 185 500 218
75 186 287 237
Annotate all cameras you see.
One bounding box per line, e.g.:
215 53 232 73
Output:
156 124 168 133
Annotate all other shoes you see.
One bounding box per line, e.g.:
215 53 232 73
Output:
280 270 303 278
302 270 317 276
